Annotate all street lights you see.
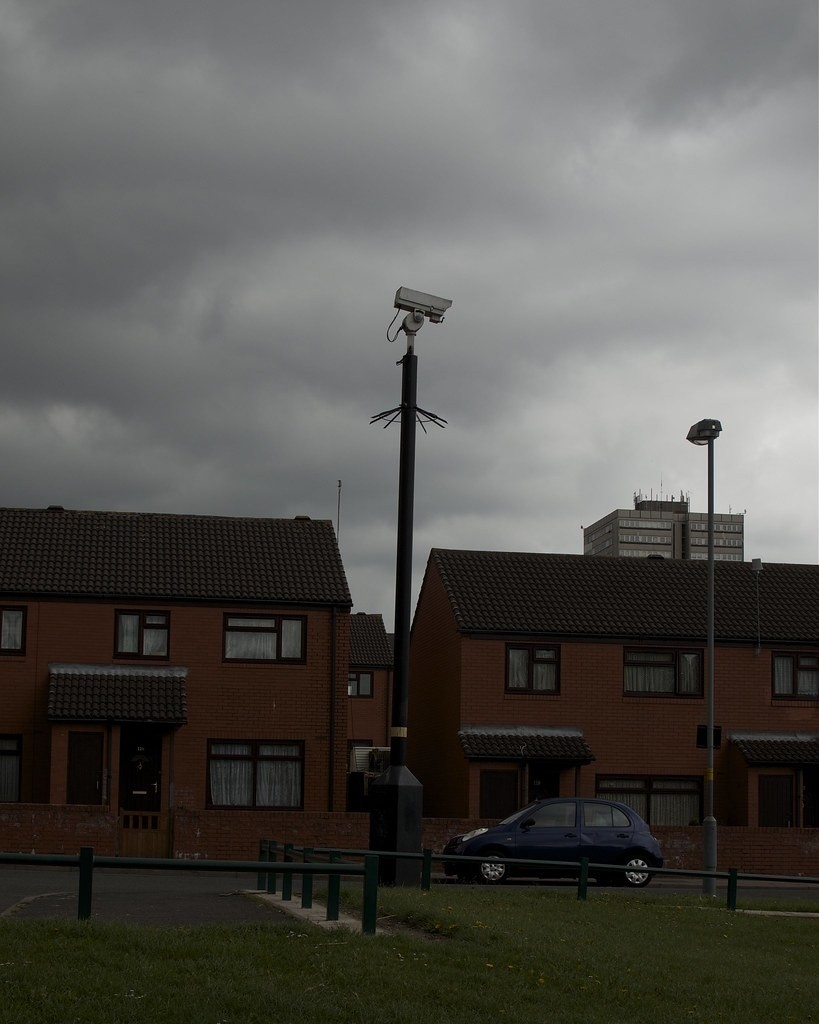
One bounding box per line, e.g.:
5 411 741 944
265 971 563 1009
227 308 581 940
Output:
684 415 724 902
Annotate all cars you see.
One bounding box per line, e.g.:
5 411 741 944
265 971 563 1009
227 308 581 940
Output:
440 797 665 886
